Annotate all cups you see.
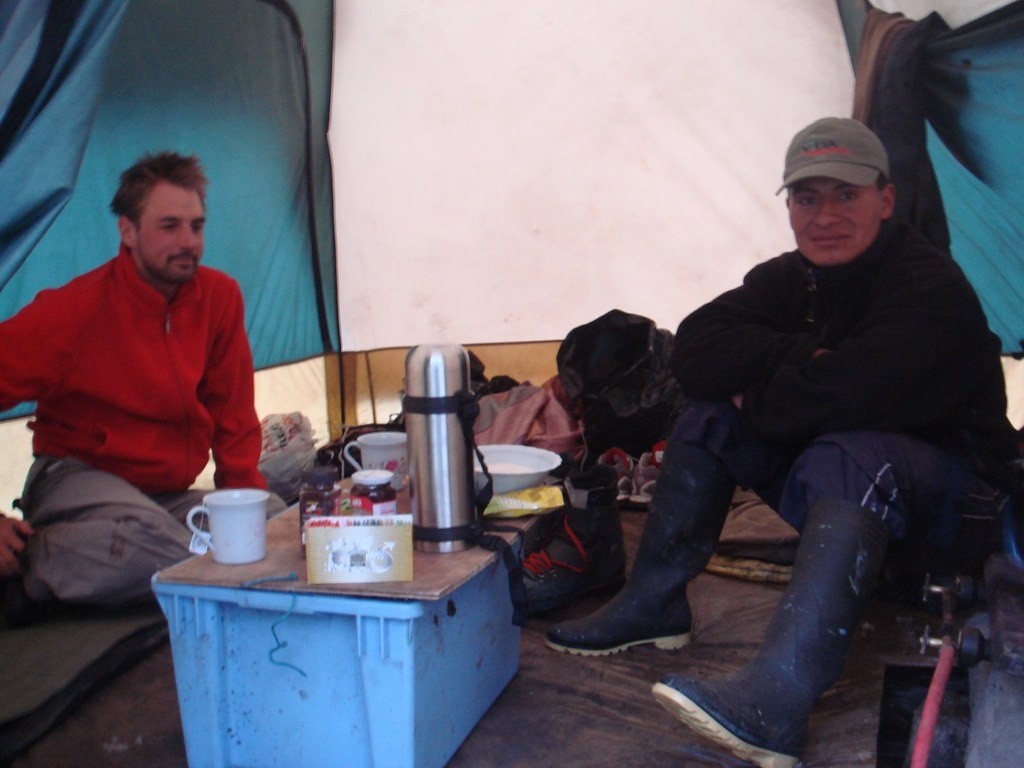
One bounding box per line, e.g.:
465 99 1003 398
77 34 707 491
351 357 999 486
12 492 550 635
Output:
345 431 410 491
186 488 270 565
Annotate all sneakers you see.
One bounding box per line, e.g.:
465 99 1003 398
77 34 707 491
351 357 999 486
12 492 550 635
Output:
629 442 667 502
598 447 640 500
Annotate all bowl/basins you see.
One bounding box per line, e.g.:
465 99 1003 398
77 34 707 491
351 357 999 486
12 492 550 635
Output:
472 444 562 495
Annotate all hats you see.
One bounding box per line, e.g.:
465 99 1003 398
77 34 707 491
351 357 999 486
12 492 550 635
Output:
774 116 892 196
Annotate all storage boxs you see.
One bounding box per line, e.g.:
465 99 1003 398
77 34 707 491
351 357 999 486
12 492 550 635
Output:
152 502 528 768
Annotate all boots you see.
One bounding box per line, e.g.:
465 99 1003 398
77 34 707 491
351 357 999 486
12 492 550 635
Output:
651 496 890 768
543 435 734 657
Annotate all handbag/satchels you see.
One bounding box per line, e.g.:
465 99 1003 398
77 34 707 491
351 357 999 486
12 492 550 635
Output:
556 308 692 459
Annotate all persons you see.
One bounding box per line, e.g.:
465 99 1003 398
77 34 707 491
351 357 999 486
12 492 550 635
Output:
545 118 1024 768
0 153 289 632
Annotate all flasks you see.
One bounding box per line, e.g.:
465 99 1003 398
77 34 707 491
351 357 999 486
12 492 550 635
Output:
400 343 475 554
